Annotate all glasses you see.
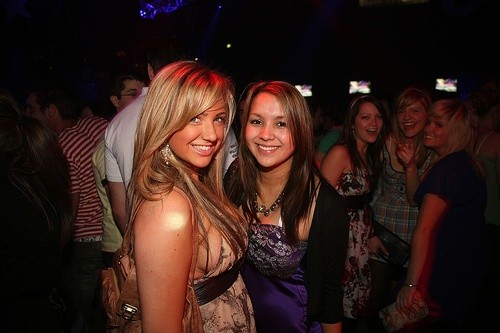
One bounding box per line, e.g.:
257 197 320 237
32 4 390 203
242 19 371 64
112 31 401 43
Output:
120 92 140 99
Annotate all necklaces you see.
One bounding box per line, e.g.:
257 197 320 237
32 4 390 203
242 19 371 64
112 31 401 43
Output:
249 166 290 216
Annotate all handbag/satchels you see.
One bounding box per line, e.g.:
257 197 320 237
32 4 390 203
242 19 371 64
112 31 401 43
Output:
95 185 199 333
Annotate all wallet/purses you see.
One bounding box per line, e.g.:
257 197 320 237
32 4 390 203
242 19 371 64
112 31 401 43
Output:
378 290 431 331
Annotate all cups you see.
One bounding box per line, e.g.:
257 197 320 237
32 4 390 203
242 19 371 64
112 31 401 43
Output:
396 138 410 156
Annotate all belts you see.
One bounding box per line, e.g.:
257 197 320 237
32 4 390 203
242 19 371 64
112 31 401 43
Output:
194 263 239 306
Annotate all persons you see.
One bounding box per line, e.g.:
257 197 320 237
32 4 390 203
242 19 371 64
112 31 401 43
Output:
371 87 434 323
395 98 500 333
24 64 500 205
319 93 390 333
116 80 344 333
24 79 114 332
122 59 260 333
0 94 73 333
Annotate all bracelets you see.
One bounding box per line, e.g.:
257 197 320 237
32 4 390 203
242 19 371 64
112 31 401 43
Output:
402 281 418 288
